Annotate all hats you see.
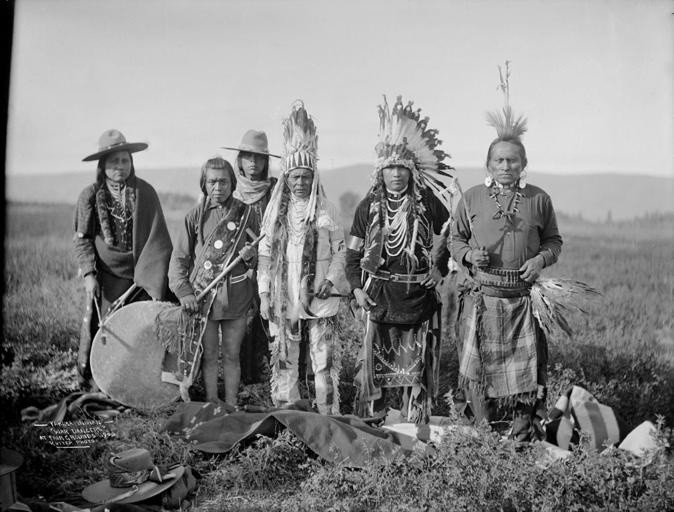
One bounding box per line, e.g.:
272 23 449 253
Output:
372 93 450 179
82 129 149 160
82 447 185 506
280 99 317 172
221 130 283 161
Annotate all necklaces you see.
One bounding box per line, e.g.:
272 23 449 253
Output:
488 187 524 222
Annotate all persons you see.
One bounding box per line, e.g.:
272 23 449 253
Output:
166 156 258 408
255 168 345 420
72 130 173 393
220 129 283 384
445 138 563 454
346 165 453 426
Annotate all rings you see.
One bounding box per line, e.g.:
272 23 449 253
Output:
322 292 327 297
